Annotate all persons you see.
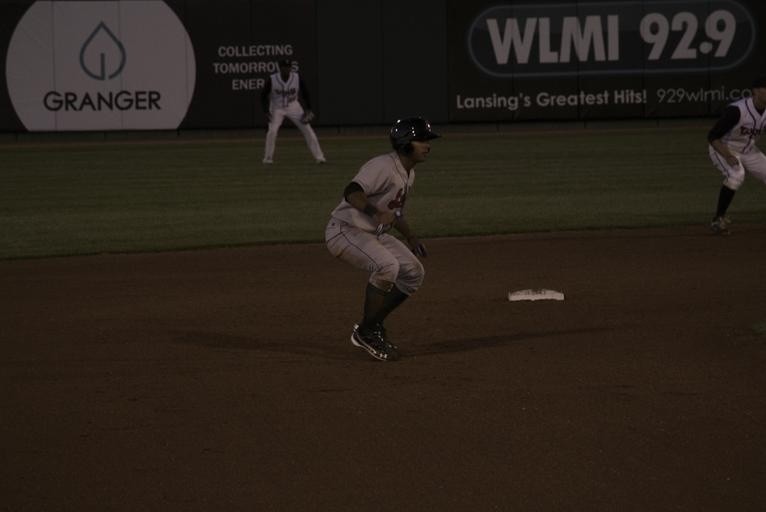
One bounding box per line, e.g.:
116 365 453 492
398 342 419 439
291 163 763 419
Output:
321 113 443 364
708 78 764 234
260 56 327 166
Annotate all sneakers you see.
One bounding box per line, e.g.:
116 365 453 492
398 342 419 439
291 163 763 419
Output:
709 218 729 235
351 322 398 361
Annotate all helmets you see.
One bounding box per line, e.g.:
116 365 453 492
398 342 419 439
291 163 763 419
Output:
390 116 441 156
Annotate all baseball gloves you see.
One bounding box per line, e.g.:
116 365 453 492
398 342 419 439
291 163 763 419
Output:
300 111 315 124
